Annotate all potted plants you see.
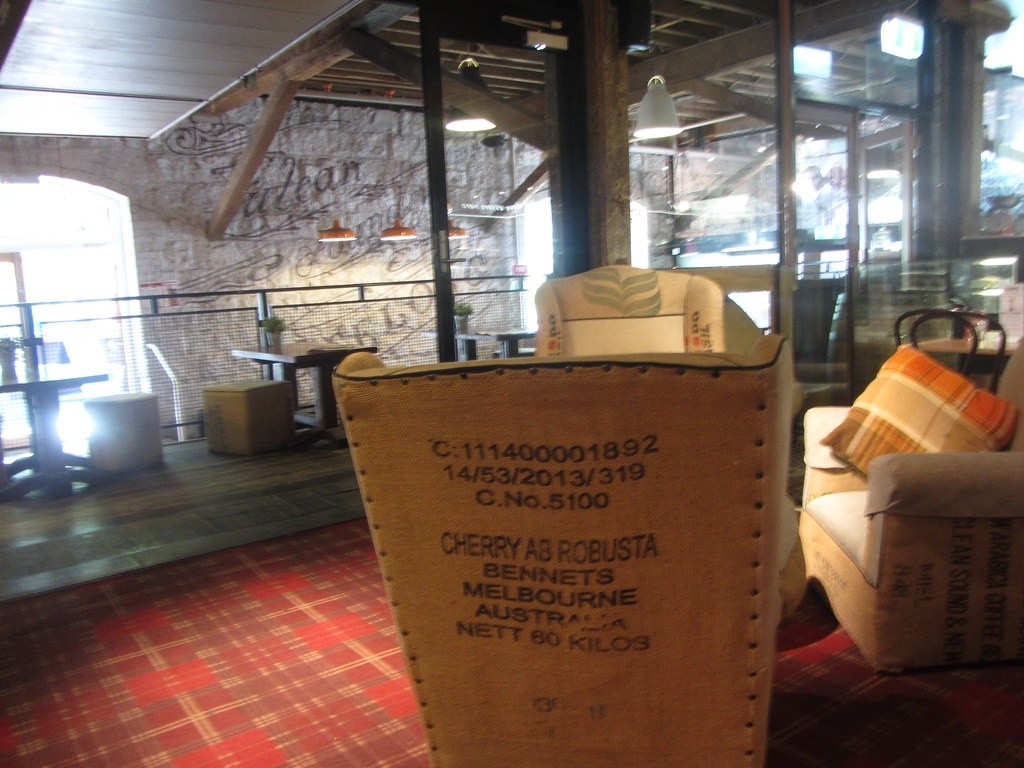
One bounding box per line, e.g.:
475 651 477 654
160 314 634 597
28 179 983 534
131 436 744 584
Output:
0 340 17 368
453 303 474 331
262 317 287 345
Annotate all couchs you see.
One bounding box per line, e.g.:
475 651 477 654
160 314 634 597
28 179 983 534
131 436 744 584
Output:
333 334 794 768
799 337 1024 674
534 267 728 355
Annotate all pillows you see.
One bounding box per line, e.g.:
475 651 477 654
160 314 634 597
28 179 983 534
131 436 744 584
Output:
820 343 1018 475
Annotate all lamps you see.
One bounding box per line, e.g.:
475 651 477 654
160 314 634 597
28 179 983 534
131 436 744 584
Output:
446 217 466 239
319 219 354 243
381 218 417 241
446 58 497 131
632 84 682 140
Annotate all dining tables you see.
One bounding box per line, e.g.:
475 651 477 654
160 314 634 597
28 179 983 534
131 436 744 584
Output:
232 343 377 450
0 362 112 502
457 330 536 359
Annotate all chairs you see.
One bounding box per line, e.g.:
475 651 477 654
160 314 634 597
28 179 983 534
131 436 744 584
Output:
892 310 1006 393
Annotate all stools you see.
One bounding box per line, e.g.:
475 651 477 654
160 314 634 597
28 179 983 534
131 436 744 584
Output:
204 381 294 456
86 392 163 471
